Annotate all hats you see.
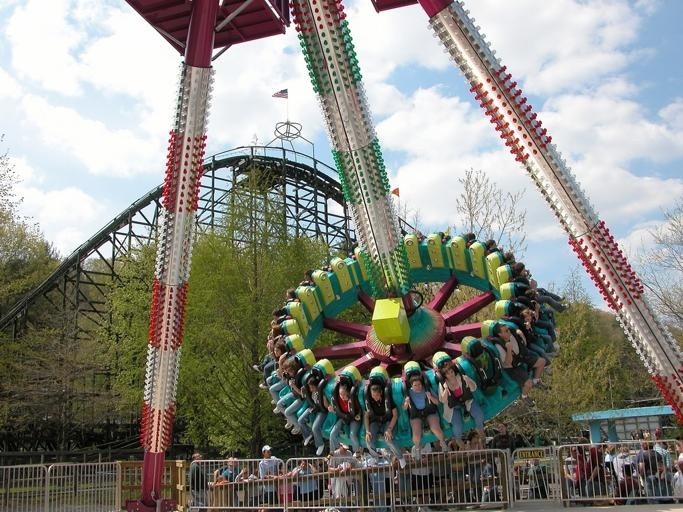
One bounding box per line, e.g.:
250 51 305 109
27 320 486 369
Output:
262 445 272 452
340 442 349 450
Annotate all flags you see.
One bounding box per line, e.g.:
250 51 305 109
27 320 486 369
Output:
270 89 288 100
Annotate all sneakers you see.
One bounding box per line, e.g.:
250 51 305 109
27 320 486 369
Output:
533 379 548 390
259 384 269 389
559 304 570 313
519 395 535 408
551 352 561 357
273 407 281 414
545 366 553 376
284 422 292 429
557 296 565 302
253 365 264 373
291 427 299 435
271 399 276 405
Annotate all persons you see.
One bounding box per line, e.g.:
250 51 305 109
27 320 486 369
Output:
251 225 570 512
187 453 211 507
212 464 229 484
234 468 257 483
219 456 239 481
570 425 682 505
